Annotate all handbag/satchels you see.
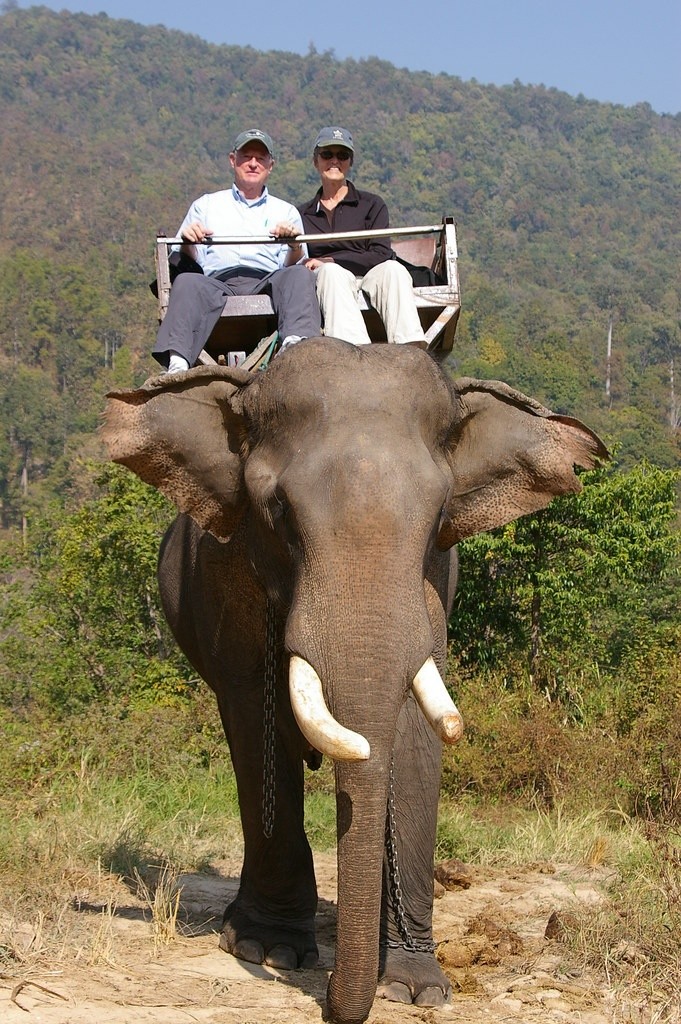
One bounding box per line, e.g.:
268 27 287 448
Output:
396 255 442 287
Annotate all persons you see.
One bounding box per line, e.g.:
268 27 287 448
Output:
150 127 324 376
296 124 430 353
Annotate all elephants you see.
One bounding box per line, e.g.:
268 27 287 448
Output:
97 335 612 1024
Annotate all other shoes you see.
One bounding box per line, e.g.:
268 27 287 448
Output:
274 333 308 359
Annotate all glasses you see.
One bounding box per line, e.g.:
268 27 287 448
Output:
318 151 350 160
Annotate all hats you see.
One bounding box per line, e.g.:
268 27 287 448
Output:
231 129 274 159
313 127 355 158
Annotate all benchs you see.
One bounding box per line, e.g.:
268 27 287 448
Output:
154 213 459 362
